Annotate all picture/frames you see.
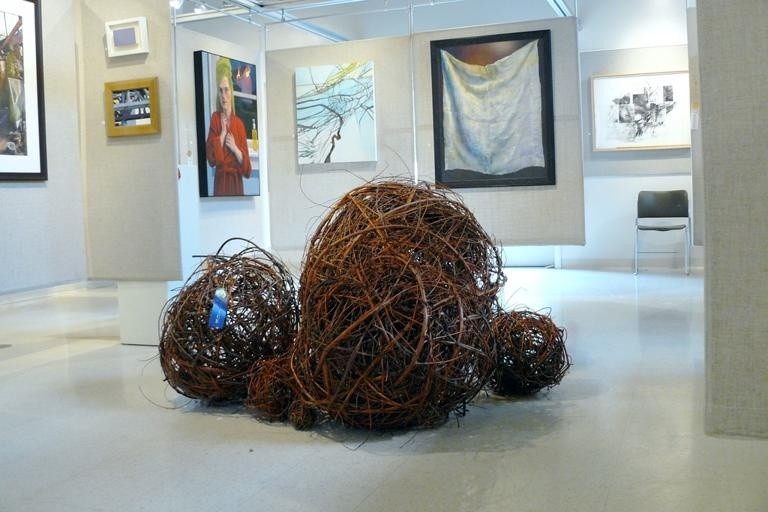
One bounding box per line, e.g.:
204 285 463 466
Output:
0 0 48 181
591 70 691 152
430 30 555 189
103 76 161 137
295 62 378 165
194 50 262 197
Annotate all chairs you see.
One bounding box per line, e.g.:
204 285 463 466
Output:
633 190 692 276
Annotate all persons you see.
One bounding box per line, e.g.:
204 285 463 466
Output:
207 58 251 197
